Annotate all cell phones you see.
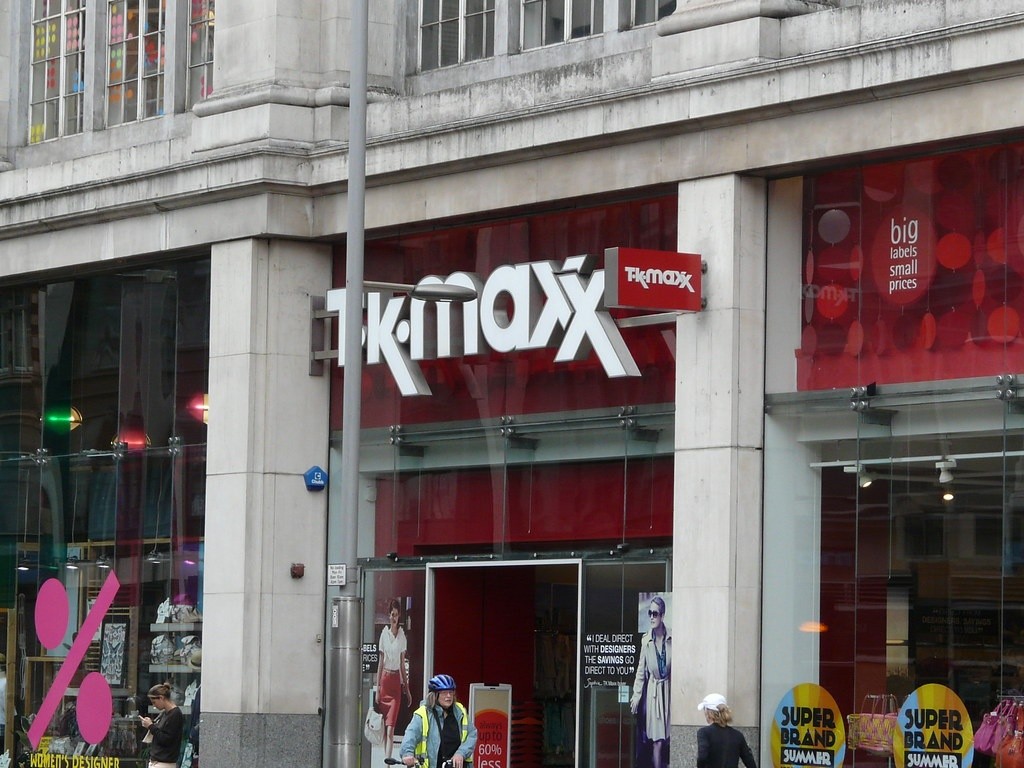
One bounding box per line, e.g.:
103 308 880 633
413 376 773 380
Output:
138 716 144 720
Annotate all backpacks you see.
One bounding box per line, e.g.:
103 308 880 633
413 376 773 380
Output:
150 634 203 706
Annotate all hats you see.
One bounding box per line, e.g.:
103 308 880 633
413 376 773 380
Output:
698 693 729 711
0 653 6 666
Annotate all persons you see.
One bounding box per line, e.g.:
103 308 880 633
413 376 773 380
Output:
375 600 413 768
138 682 183 768
697 692 757 768
400 674 478 767
630 597 672 768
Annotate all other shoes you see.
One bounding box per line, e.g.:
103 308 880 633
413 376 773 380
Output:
171 606 202 622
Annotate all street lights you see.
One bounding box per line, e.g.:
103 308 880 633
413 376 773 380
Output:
320 279 483 768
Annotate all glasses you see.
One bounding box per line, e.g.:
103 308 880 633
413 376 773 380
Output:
648 610 660 618
439 691 454 695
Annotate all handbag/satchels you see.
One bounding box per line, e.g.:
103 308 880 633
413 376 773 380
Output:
974 700 1024 768
142 710 163 743
364 701 384 745
847 695 899 752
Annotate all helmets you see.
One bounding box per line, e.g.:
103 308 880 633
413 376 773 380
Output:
428 675 456 692
